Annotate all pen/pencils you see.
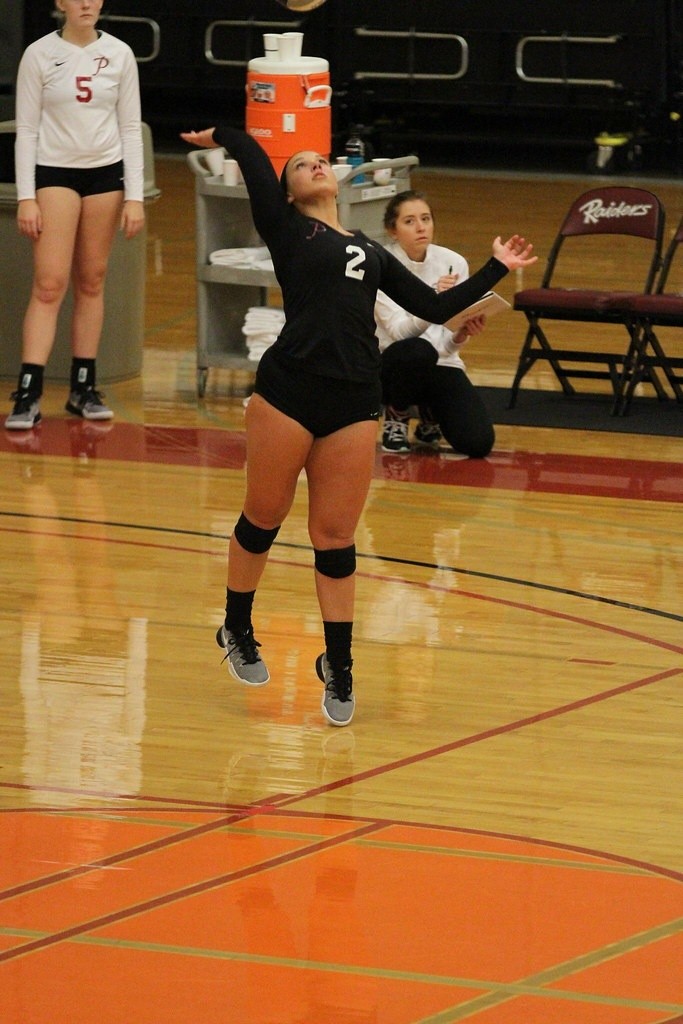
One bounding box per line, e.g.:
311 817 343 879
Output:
448 265 453 274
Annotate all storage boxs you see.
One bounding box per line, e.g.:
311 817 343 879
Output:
0 116 162 388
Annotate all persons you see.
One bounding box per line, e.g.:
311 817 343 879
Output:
5 0 145 428
180 127 538 727
374 191 495 459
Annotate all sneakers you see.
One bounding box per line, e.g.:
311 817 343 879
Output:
381 406 444 455
214 627 270 686
6 401 42 429
316 653 355 726
65 393 114 420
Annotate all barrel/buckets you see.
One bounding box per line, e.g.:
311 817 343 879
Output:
246 57 331 183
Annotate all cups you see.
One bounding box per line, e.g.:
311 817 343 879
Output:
332 165 351 187
204 149 225 176
285 33 304 57
263 33 281 60
373 159 392 185
337 157 347 164
223 161 239 186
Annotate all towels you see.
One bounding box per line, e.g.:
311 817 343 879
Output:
208 245 275 272
240 305 286 361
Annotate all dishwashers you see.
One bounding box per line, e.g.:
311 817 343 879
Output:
277 37 297 62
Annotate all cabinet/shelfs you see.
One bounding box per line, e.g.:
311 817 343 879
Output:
194 174 410 373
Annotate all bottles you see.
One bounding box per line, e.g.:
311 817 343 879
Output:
346 133 364 183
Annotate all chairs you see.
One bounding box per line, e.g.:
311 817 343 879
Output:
618 215 683 418
507 185 668 419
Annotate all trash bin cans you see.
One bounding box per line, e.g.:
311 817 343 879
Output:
0 117 162 385
